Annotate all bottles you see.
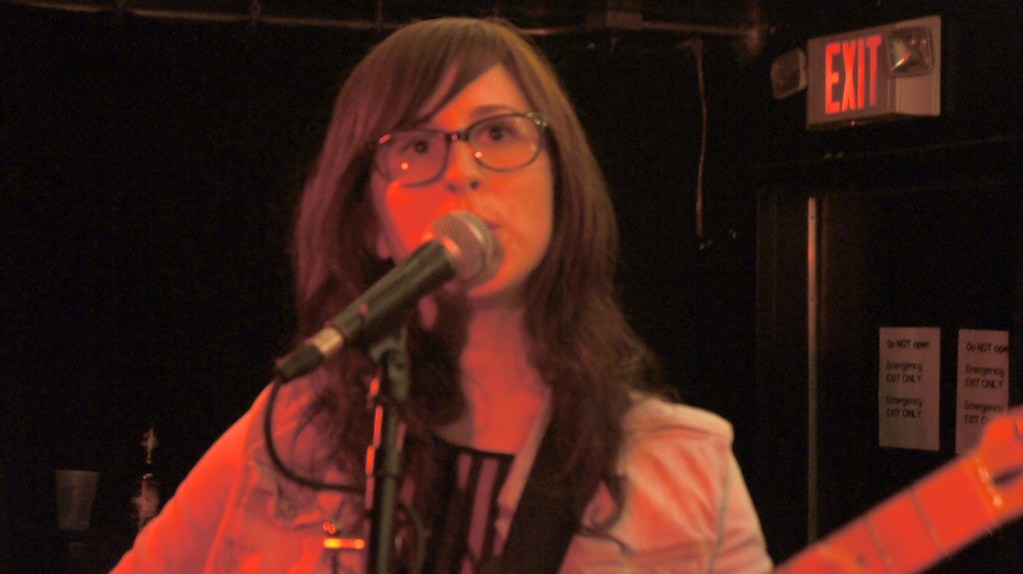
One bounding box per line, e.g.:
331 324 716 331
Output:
125 420 161 548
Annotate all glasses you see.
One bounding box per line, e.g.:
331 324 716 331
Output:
372 111 554 189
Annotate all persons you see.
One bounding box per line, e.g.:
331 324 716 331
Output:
106 17 778 574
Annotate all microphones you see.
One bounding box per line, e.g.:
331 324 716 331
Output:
274 210 493 384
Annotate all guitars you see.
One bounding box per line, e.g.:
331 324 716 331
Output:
776 410 1022 574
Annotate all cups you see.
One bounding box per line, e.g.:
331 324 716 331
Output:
54 469 100 535
